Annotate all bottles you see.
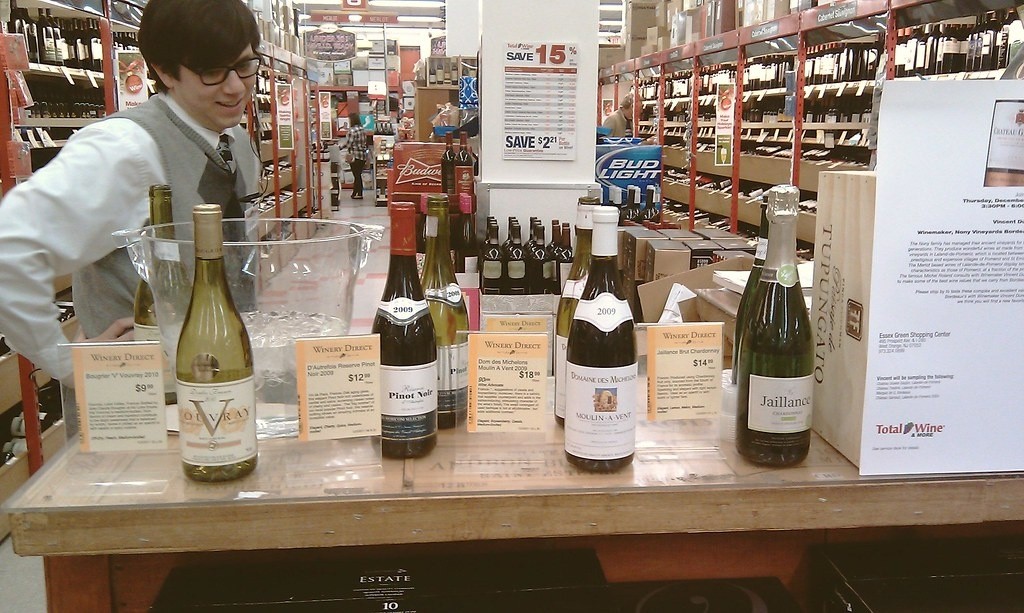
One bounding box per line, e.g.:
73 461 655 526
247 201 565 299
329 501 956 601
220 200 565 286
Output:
735 184 816 469
133 183 193 405
732 195 769 386
639 8 1019 256
563 207 639 473
372 201 438 459
456 197 474 273
419 193 471 430
374 122 394 135
440 132 455 194
8 0 138 119
176 205 259 484
601 184 659 226
477 216 574 295
454 132 479 193
553 197 602 427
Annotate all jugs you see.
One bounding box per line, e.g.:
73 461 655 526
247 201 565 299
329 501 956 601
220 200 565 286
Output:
111 218 386 440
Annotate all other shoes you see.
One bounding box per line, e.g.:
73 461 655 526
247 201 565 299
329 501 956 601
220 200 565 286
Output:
350 195 354 199
354 196 363 199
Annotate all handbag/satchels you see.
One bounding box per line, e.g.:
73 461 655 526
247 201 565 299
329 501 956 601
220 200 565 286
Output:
345 154 354 163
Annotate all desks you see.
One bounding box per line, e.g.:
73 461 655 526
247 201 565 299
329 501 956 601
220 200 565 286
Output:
0 367 1024 613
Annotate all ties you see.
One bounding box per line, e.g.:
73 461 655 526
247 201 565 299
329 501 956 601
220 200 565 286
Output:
217 132 235 177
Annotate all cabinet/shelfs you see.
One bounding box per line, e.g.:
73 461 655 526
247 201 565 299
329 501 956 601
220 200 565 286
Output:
597 0 1023 264
414 55 478 142
597 0 626 47
0 0 321 545
299 10 403 189
373 135 395 208
312 153 341 212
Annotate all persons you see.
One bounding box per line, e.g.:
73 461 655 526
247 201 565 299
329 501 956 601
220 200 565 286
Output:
338 113 367 199
603 93 658 145
0 0 261 392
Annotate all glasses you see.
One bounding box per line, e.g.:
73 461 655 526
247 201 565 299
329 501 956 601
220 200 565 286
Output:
179 49 262 85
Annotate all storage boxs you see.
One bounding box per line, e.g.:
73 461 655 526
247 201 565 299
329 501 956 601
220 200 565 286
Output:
598 0 845 71
617 225 757 282
318 41 400 86
149 535 1024 613
336 102 375 132
243 0 305 57
